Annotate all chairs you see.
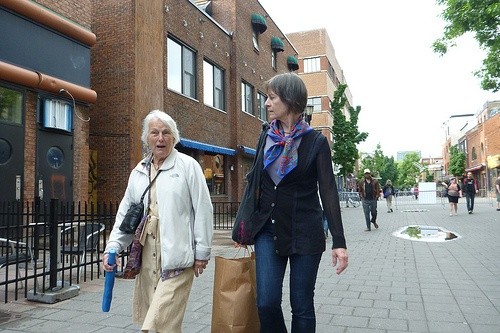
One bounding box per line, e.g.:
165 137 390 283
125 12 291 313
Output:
0 222 105 288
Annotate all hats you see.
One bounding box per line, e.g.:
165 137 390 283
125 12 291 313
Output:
364 169 371 174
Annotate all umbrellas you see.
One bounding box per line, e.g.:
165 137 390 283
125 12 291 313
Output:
102 249 116 312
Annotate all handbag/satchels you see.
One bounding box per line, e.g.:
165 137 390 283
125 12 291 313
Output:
119 202 144 235
233 122 270 246
211 244 261 333
123 217 146 279
384 187 391 197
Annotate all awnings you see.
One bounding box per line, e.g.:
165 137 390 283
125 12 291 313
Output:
288 56 299 71
251 12 267 34
236 145 256 157
179 137 235 156
272 36 284 52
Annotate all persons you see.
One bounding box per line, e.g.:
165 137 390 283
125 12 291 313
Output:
234 73 348 333
344 183 382 208
317 181 328 238
102 110 214 333
383 180 393 213
495 174 500 211
463 172 479 214
395 186 419 200
359 169 379 231
442 177 462 216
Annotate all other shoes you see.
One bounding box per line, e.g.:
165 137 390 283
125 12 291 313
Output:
497 209 500 211
390 209 393 212
388 211 390 213
455 211 458 216
372 221 378 228
469 210 473 214
450 213 453 216
367 224 371 231
